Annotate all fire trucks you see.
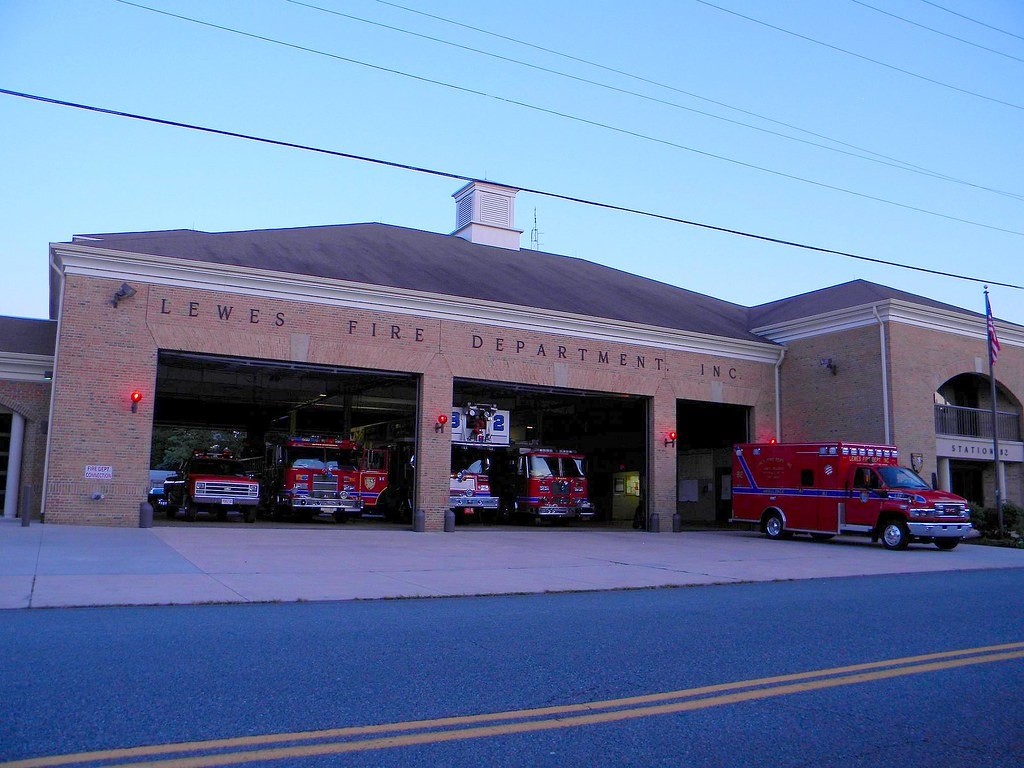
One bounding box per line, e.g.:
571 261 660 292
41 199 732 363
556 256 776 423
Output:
726 438 973 551
492 438 597 527
164 445 261 523
245 430 391 523
349 402 510 526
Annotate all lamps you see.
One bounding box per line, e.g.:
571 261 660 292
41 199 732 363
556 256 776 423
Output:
44 370 53 380
819 358 837 376
131 390 143 413
434 414 448 433
664 432 678 448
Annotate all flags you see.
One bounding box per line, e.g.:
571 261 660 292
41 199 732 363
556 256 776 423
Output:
986 294 1001 367
